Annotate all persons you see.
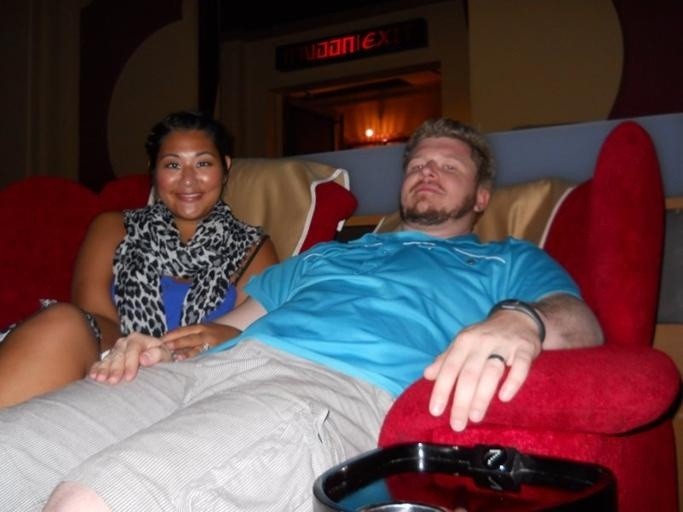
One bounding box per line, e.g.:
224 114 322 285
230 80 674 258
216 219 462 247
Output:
0 116 607 512
0 106 283 410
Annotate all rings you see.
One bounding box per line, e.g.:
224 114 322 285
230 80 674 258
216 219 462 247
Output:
486 353 509 370
202 344 211 352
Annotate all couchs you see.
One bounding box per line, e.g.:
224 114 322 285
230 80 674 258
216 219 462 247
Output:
1 121 682 509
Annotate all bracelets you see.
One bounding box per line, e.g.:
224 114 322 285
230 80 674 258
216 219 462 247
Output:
489 297 546 344
